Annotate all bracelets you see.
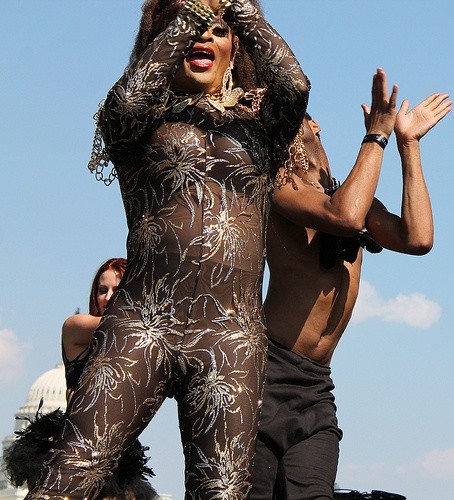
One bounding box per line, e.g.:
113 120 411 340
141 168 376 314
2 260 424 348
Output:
362 131 389 151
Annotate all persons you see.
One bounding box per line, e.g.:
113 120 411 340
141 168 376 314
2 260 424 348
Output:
64 258 150 498
228 64 451 500
24 0 313 500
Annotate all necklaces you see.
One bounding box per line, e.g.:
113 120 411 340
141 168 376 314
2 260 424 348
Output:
163 74 229 106
